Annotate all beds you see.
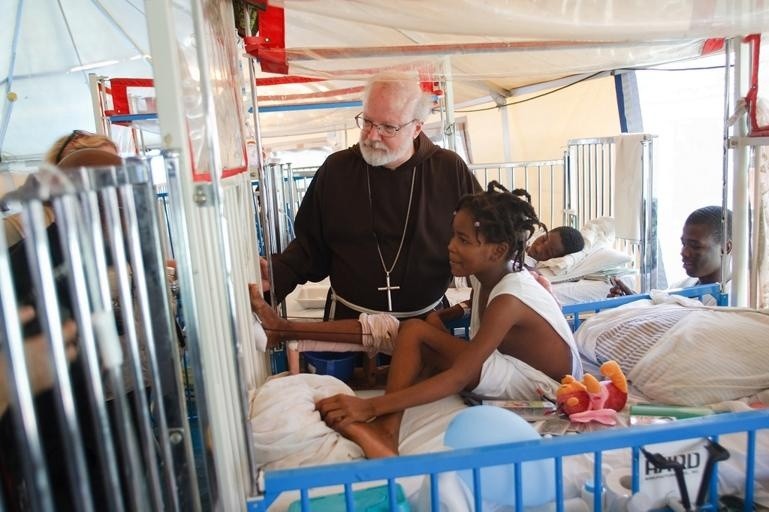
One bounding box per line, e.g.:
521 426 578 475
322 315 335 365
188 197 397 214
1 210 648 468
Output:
275 255 639 373
249 282 768 512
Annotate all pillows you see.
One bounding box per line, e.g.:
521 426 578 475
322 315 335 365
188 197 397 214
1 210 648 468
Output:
534 216 637 285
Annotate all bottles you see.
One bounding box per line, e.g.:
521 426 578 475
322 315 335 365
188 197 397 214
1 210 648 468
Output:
581 482 606 510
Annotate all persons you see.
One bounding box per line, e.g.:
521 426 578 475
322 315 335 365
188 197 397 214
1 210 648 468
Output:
250 180 584 460
44 132 123 169
259 67 488 373
604 205 733 297
0 306 79 420
446 225 584 287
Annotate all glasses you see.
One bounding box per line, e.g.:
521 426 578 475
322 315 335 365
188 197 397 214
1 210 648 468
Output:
354 112 416 137
54 130 95 163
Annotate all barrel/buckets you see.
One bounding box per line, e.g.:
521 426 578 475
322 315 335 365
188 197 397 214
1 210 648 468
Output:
302 350 356 384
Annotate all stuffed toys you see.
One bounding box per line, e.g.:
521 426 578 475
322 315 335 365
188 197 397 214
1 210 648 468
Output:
555 359 628 426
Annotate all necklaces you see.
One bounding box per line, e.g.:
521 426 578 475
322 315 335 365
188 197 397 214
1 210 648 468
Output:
363 167 418 313
